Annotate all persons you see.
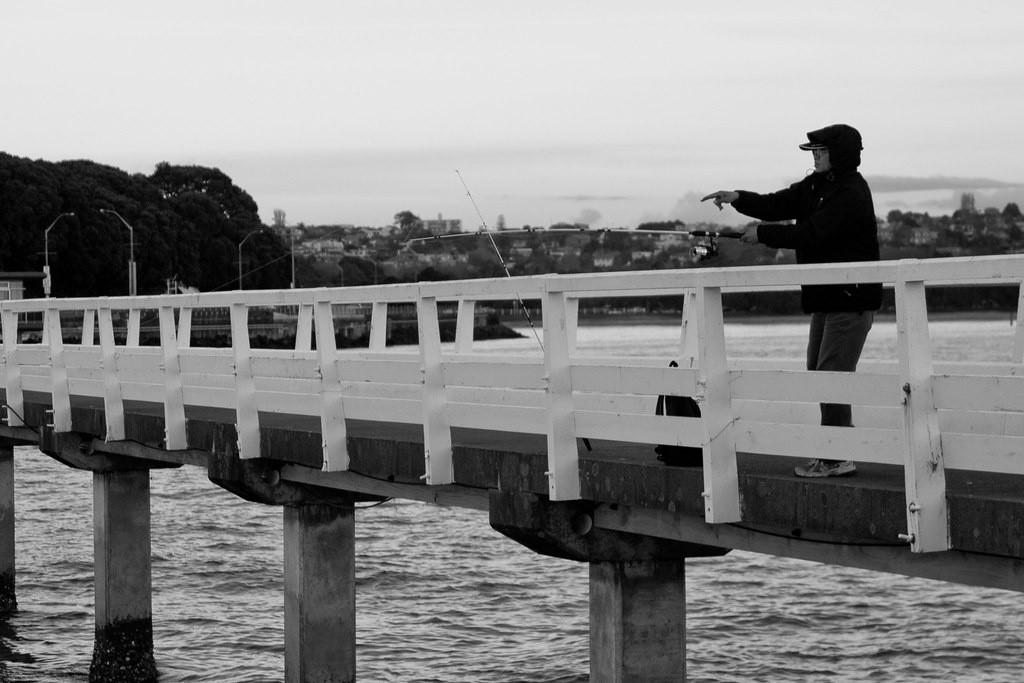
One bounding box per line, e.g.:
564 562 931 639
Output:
701 123 883 476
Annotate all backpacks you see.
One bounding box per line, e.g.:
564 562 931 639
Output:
654 361 703 468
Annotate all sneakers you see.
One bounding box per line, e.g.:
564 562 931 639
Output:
793 459 856 477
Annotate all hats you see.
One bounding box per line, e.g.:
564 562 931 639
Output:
799 140 828 150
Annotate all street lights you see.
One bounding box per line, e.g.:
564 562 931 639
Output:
273 218 295 288
101 208 136 295
239 231 255 289
44 213 74 298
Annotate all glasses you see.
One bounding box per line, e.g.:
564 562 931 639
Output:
812 149 829 157
